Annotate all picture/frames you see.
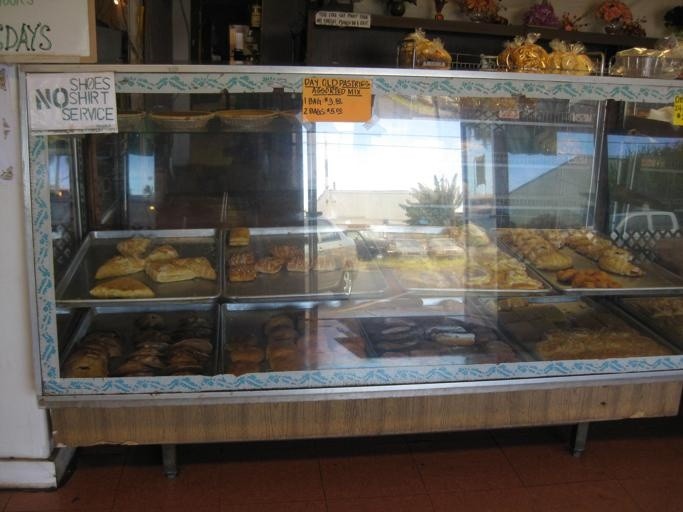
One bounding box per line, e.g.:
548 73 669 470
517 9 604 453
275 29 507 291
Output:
0 0 98 65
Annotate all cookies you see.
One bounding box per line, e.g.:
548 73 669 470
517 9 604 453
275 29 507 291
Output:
497 44 594 78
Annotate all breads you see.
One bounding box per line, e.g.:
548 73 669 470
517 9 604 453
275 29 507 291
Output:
227 303 367 374
61 313 216 376
364 296 522 366
89 234 216 299
486 297 674 358
623 297 682 350
383 222 544 289
494 228 646 287
229 227 366 281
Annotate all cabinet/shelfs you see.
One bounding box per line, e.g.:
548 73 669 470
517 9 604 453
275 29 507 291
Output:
16 62 683 485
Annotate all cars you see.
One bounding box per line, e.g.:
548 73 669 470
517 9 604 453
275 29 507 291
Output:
304 217 461 299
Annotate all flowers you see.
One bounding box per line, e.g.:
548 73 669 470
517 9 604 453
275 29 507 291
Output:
467 0 632 32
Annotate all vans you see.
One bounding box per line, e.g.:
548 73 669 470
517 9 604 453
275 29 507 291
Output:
611 211 680 261
